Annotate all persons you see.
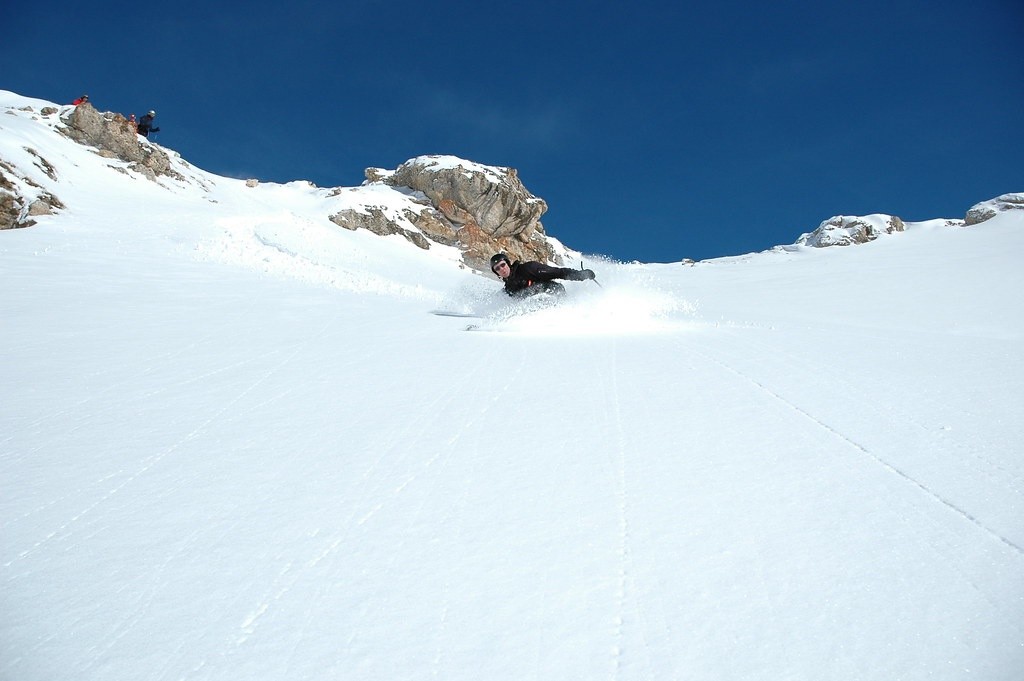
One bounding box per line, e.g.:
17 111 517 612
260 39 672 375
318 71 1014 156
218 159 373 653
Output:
73 95 161 138
490 254 596 307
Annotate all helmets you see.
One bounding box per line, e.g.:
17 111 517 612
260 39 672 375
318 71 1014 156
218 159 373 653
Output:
490 254 510 279
148 110 155 117
84 95 88 99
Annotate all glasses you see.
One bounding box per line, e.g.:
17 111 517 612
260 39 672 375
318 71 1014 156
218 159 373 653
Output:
495 263 506 271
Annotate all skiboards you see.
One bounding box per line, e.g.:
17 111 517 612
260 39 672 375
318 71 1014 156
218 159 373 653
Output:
431 310 486 332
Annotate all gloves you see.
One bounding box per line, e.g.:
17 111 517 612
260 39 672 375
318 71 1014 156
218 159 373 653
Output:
577 269 595 281
